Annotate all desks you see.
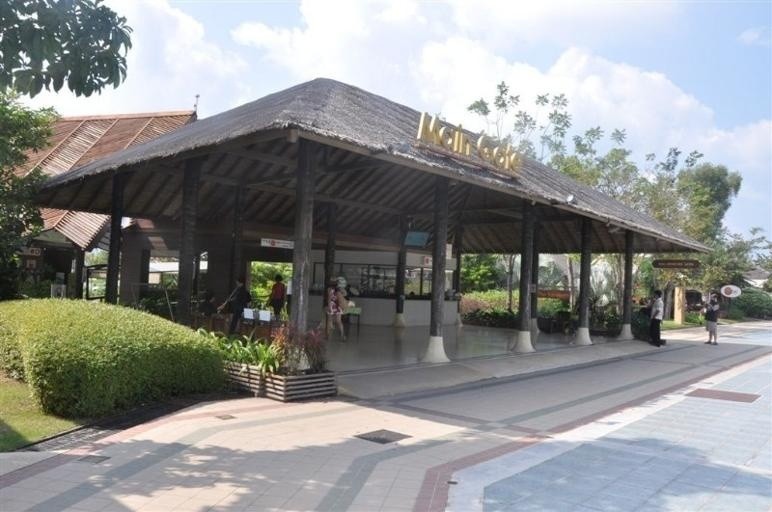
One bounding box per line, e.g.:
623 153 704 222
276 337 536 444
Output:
324 307 361 337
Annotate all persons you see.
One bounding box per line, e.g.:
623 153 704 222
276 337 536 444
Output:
192 275 199 302
699 294 721 346
326 275 350 343
227 275 253 338
648 289 665 348
198 290 226 317
265 273 287 320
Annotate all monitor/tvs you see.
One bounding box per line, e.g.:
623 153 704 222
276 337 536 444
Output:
403 230 430 247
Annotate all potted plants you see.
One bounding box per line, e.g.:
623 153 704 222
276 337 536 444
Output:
222 332 336 402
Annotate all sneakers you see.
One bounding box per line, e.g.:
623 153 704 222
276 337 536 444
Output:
704 340 717 344
